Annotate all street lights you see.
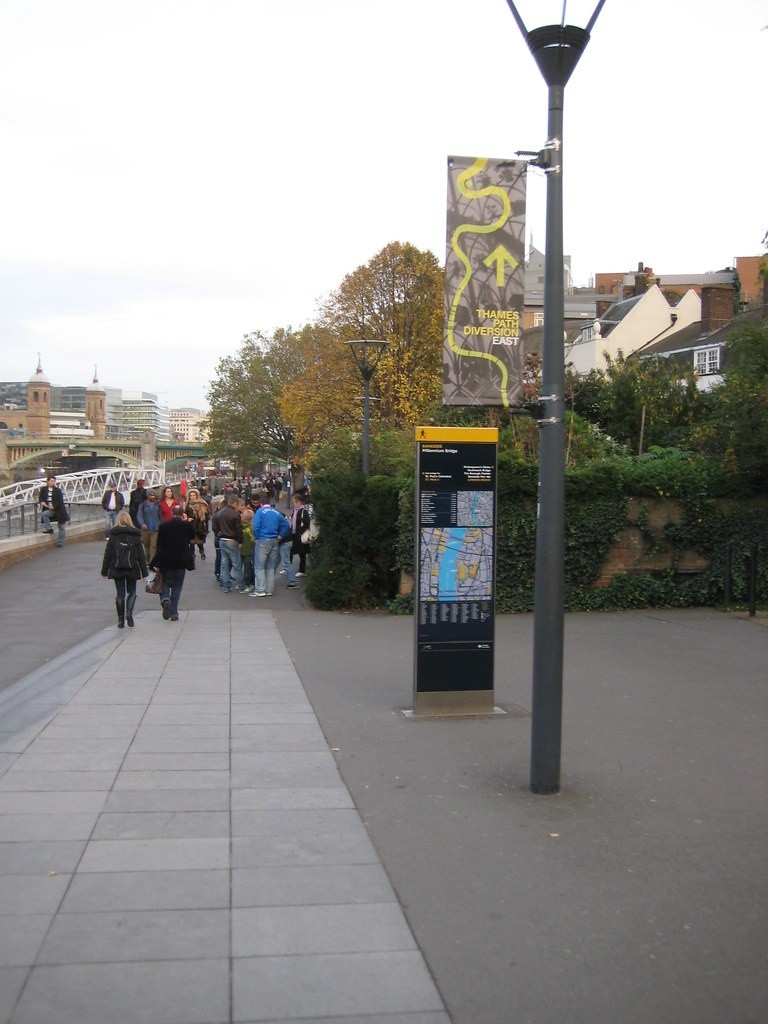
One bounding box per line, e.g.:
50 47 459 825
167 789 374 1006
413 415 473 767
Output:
344 338 391 475
506 0 604 793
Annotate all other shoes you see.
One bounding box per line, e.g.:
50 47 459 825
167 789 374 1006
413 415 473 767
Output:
296 572 307 577
42 529 53 533
286 584 300 588
215 574 272 597
162 600 172 619
106 537 109 541
279 568 287 574
171 617 179 621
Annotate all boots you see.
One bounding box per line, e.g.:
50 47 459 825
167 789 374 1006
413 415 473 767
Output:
126 595 135 627
116 598 125 628
199 548 206 560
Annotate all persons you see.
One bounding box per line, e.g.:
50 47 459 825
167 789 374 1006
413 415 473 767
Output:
102 483 124 540
38 476 69 548
100 511 148 628
139 473 314 597
129 479 147 529
148 504 196 621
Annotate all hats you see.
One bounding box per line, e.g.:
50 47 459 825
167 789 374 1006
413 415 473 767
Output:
146 488 157 497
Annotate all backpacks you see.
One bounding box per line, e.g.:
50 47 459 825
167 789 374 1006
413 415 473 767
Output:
111 533 137 569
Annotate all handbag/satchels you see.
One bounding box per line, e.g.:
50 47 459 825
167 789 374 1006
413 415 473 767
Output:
301 508 320 544
145 570 163 593
